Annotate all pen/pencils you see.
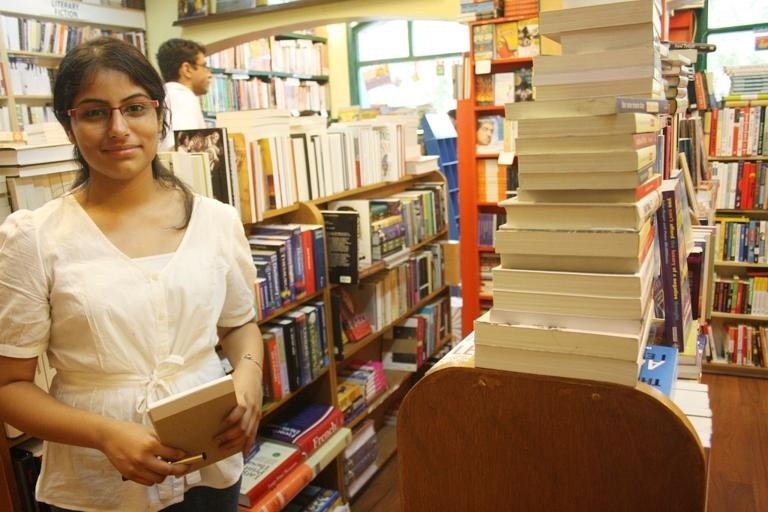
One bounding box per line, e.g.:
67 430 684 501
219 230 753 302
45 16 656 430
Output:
121 452 207 481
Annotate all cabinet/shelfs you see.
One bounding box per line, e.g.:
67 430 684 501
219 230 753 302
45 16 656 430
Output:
199 37 330 118
397 310 709 512
0 113 460 512
691 98 766 378
2 12 151 130
459 20 536 338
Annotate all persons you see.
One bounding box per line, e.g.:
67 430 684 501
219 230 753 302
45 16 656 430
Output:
156 38 212 145
1 37 264 512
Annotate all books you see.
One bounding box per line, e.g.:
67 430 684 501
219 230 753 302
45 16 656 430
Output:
703 66 767 371
203 27 328 114
157 107 458 512
0 350 58 439
1 0 147 237
455 0 718 511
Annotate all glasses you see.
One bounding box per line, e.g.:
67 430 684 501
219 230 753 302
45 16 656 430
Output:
67 100 159 124
